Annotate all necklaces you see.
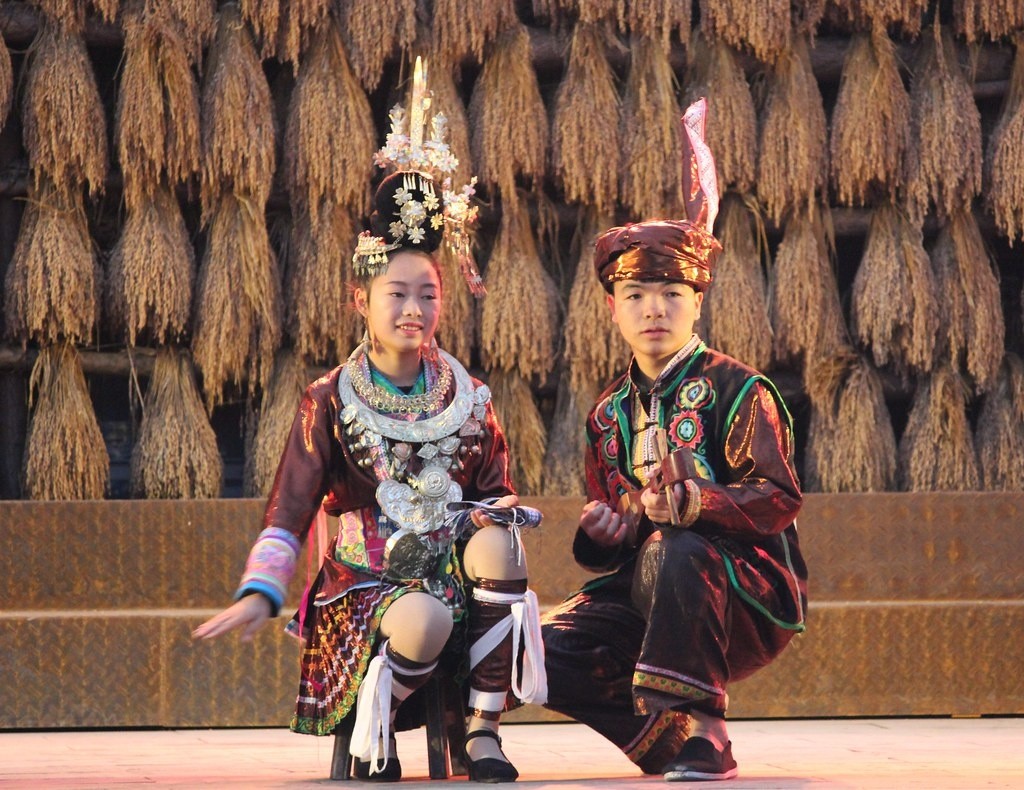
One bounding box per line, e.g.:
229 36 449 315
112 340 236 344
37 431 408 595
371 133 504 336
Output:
337 339 490 590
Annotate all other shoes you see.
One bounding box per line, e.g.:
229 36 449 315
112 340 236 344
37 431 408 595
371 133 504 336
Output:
354 732 401 782
458 730 520 784
663 736 738 782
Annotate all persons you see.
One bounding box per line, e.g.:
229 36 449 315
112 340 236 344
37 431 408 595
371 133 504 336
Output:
192 171 529 783
524 221 812 781
